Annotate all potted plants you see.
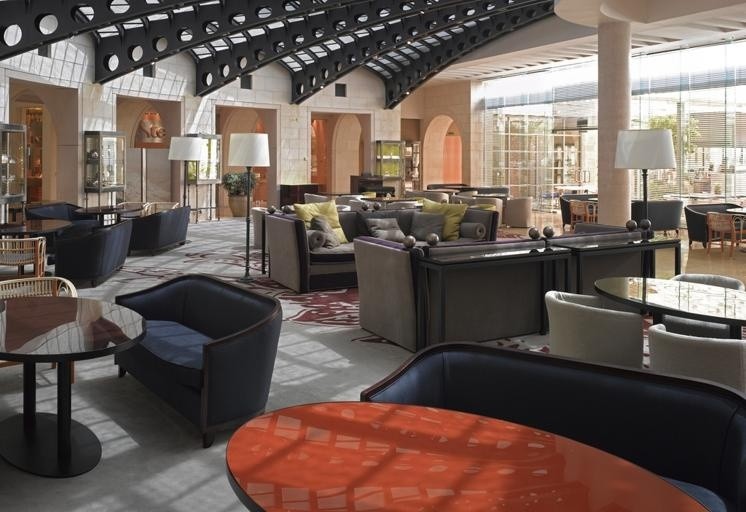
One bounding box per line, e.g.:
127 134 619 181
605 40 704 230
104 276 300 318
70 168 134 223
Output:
224 172 257 217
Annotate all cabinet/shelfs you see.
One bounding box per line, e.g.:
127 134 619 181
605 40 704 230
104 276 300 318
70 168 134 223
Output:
405 140 421 182
0 121 27 222
375 140 405 192
83 131 125 207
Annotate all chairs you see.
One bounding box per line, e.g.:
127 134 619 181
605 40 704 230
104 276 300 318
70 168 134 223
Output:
0 276 78 384
0 201 191 287
648 323 746 397
545 291 644 368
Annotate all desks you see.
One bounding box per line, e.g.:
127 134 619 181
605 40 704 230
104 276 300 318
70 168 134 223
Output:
226 403 708 512
0 296 146 478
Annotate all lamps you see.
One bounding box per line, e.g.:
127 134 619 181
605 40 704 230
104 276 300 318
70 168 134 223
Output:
615 129 677 219
228 132 271 284
168 136 203 209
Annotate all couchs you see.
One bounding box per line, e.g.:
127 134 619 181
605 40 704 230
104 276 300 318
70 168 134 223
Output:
116 273 282 447
362 338 746 512
